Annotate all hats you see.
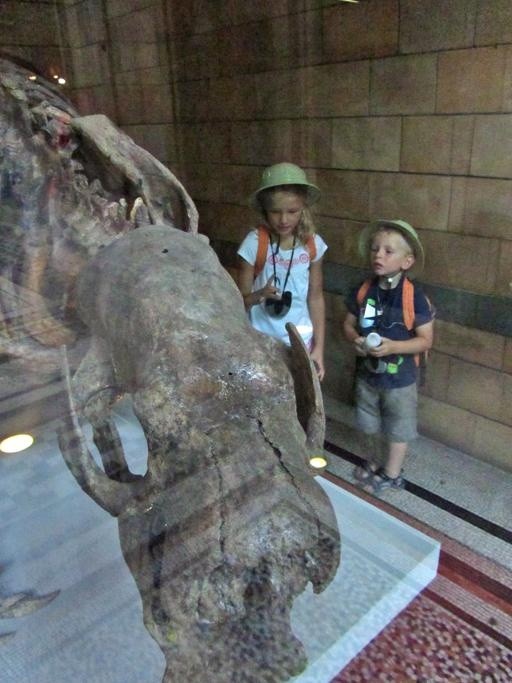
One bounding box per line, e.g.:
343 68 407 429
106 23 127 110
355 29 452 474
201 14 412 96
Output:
249 162 321 214
357 218 425 283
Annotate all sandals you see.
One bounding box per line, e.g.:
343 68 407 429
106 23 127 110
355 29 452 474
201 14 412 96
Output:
354 457 406 494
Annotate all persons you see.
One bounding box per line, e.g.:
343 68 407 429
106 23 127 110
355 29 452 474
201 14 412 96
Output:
339 217 438 498
234 160 331 382
